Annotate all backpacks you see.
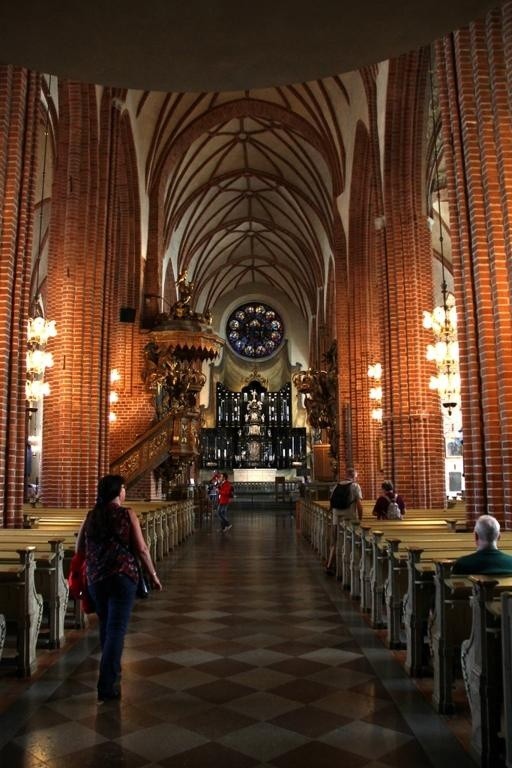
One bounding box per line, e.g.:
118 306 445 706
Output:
330 482 356 509
380 494 403 520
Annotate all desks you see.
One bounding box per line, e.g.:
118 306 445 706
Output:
0 498 195 682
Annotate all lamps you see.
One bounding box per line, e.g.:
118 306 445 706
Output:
422 303 462 418
109 368 120 423
366 362 382 424
23 294 58 420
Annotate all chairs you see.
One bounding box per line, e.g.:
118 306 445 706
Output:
276 475 292 505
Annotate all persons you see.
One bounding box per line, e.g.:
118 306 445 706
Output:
175 268 192 305
70 475 164 701
373 479 405 520
210 472 221 501
445 515 511 575
319 468 362 577
216 474 235 533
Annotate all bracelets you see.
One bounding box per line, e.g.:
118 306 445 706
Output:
149 572 158 576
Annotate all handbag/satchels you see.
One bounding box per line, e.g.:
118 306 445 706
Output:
137 570 148 600
